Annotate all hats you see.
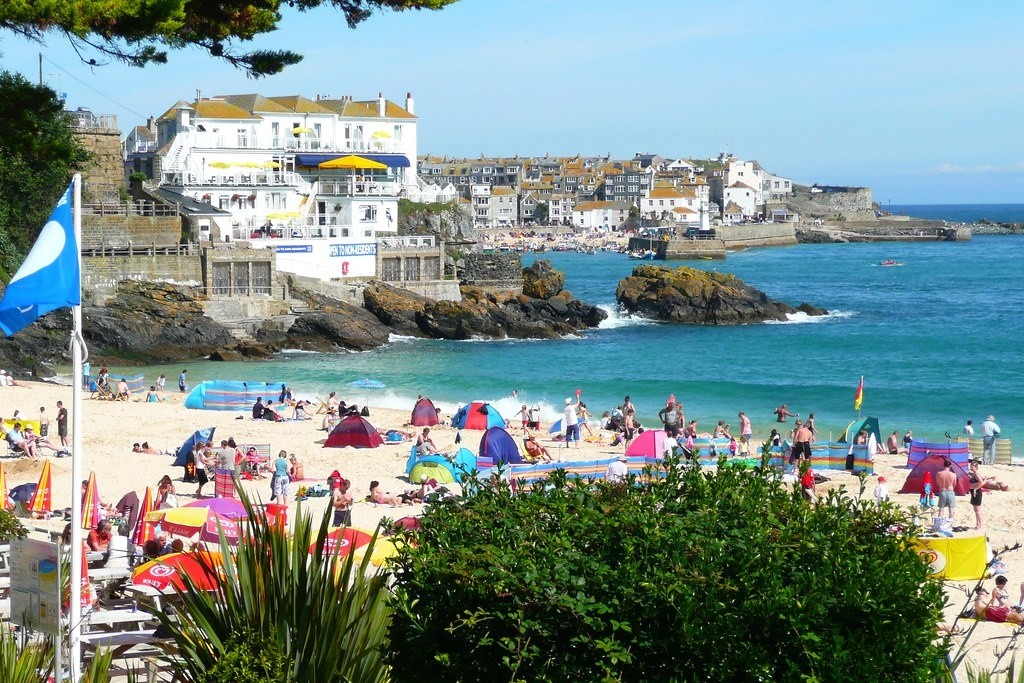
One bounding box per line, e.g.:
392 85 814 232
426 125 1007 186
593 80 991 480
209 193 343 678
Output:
24 424 34 431
977 588 990 595
995 576 1008 584
564 397 572 405
987 415 995 421
877 476 886 482
420 474 428 483
171 538 183 551
529 433 535 439
616 455 627 463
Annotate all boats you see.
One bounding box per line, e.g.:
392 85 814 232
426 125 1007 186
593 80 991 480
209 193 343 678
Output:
483 241 610 255
616 248 656 260
882 261 895 265
699 255 712 260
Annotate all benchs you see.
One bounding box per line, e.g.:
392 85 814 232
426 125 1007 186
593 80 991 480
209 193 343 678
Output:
81 650 189 676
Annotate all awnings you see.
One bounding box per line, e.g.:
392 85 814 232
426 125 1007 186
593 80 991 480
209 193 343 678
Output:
294 154 411 168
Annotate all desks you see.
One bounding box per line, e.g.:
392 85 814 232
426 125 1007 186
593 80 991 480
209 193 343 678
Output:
79 628 174 661
90 606 153 632
88 566 133 592
62 551 112 569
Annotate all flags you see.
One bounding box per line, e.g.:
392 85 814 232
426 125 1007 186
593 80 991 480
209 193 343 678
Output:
854 378 864 411
0 181 80 336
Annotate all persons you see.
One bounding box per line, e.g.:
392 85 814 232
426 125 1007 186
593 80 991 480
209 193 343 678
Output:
0 361 1024 683
770 190 790 204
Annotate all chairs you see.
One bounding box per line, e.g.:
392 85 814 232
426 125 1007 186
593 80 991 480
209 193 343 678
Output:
521 440 546 465
5 431 45 459
316 396 339 415
90 377 127 402
191 446 271 479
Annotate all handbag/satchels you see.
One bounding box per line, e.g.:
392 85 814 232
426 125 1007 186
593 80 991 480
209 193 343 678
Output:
118 518 130 536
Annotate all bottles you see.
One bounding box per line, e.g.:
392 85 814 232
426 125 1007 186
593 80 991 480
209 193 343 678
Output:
132 600 137 613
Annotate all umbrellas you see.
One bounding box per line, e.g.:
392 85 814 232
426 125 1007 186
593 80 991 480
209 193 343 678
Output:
136 507 252 548
340 534 423 569
207 159 304 228
179 499 276 526
0 461 7 510
317 155 388 197
293 526 387 559
133 548 241 596
81 471 101 529
27 458 50 514
293 126 392 152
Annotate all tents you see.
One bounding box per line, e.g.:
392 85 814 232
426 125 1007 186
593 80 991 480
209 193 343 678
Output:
322 414 383 448
412 398 439 426
453 402 507 430
897 454 970 496
480 426 521 465
625 429 672 458
407 445 477 484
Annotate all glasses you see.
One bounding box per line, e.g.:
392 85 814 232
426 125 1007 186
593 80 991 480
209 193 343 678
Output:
201 446 205 448
250 451 254 453
104 527 111 532
797 423 802 426
1001 583 1007 586
